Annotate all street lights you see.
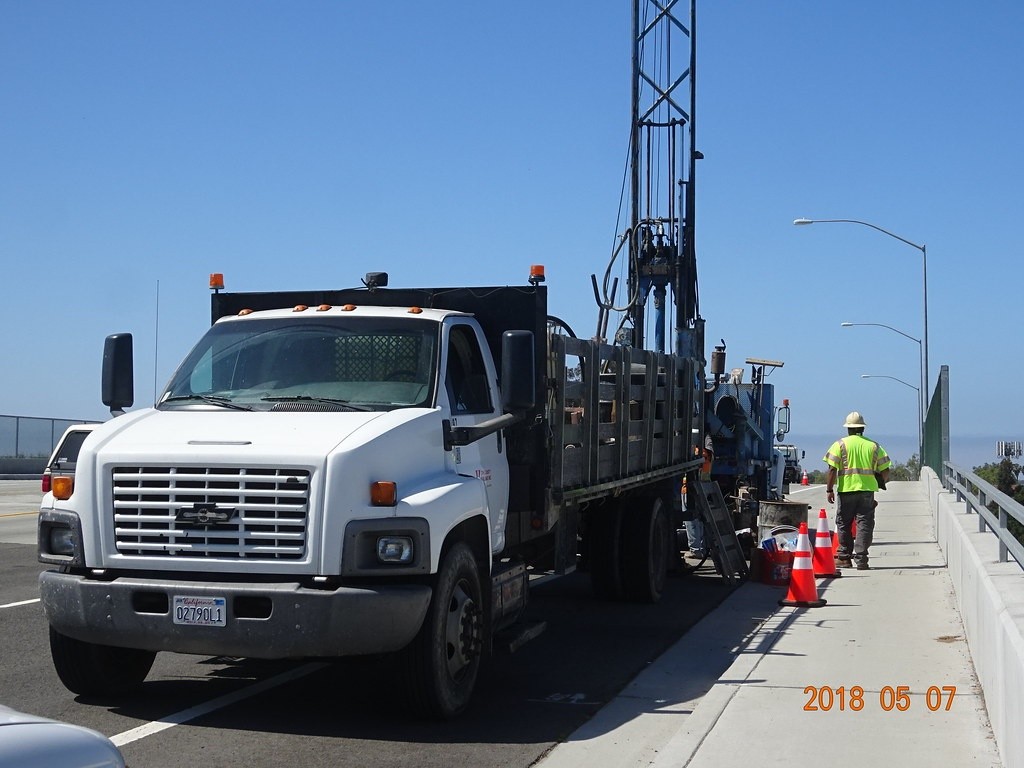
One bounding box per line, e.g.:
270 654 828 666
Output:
841 322 925 424
860 374 921 448
793 218 930 413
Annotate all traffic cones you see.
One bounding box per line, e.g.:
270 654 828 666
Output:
776 520 828 609
800 468 810 486
812 508 843 580
850 516 858 538
830 524 841 559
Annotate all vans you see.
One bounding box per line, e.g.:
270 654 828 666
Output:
41 422 104 494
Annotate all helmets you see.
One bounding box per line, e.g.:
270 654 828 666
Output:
842 412 867 427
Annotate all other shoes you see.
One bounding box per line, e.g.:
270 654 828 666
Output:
857 563 869 569
835 559 852 568
685 551 702 559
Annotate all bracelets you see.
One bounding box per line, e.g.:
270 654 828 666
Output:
826 489 833 492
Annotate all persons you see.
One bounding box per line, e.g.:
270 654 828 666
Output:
823 412 892 571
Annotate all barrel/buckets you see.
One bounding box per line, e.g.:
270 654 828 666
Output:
759 551 795 585
757 500 809 547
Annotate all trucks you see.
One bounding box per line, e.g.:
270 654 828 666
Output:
31 262 708 723
677 336 807 544
